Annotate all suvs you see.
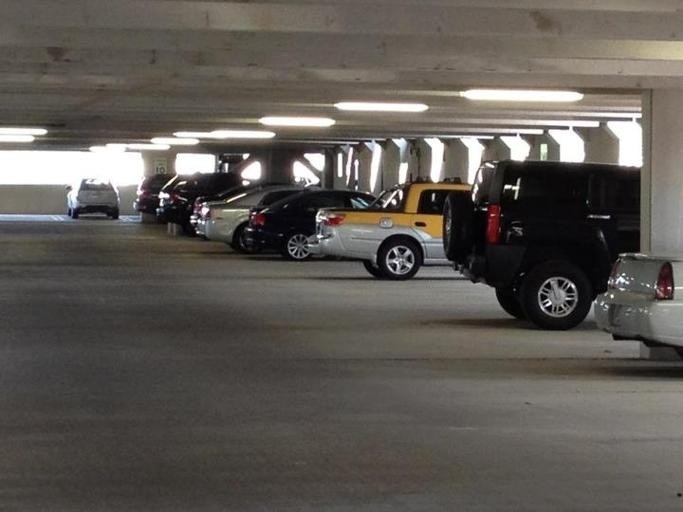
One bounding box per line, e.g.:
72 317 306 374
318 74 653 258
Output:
62 176 122 224
440 157 641 330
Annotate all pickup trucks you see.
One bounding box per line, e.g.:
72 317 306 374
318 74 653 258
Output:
313 172 471 281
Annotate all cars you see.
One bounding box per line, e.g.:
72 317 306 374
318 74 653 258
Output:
590 250 682 354
133 169 390 264
